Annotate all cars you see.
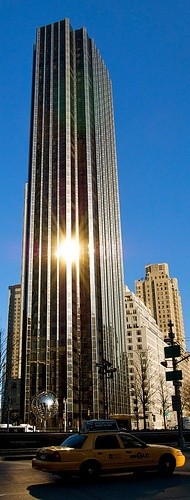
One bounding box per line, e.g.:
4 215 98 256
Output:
32 431 185 480
